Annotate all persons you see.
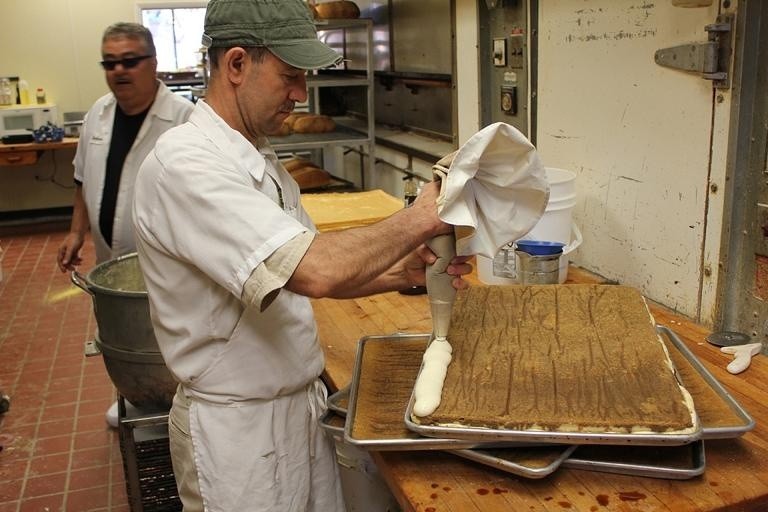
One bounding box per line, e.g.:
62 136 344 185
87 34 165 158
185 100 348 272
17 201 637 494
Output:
57 24 194 434
133 0 476 512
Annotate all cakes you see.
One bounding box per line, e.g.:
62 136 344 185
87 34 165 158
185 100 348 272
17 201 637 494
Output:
410 283 697 435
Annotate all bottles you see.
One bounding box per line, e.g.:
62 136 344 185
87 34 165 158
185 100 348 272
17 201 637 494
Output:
402 181 419 210
17 76 34 106
0 78 14 106
35 88 47 104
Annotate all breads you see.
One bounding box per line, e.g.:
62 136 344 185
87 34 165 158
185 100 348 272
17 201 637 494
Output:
315 1 360 19
282 159 330 188
273 111 337 136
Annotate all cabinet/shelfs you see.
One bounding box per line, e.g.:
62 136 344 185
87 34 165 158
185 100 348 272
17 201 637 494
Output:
261 12 375 196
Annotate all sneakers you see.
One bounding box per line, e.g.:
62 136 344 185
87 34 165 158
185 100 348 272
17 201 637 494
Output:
104 397 143 428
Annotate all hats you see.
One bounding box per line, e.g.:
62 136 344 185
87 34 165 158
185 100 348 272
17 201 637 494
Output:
201 1 345 71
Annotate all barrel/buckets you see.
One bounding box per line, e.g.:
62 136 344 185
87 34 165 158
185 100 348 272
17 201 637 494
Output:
477 168 582 283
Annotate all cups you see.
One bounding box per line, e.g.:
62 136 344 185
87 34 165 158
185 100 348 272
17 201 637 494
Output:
515 247 561 285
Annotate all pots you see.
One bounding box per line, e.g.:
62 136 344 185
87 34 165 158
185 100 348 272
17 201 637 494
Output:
67 247 179 412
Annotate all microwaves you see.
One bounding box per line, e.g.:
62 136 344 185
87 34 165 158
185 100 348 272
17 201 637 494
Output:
0 104 64 140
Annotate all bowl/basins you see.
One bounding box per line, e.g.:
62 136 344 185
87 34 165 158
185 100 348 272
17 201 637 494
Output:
514 238 568 257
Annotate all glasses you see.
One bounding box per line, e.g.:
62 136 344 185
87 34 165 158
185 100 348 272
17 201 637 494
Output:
101 56 153 71
